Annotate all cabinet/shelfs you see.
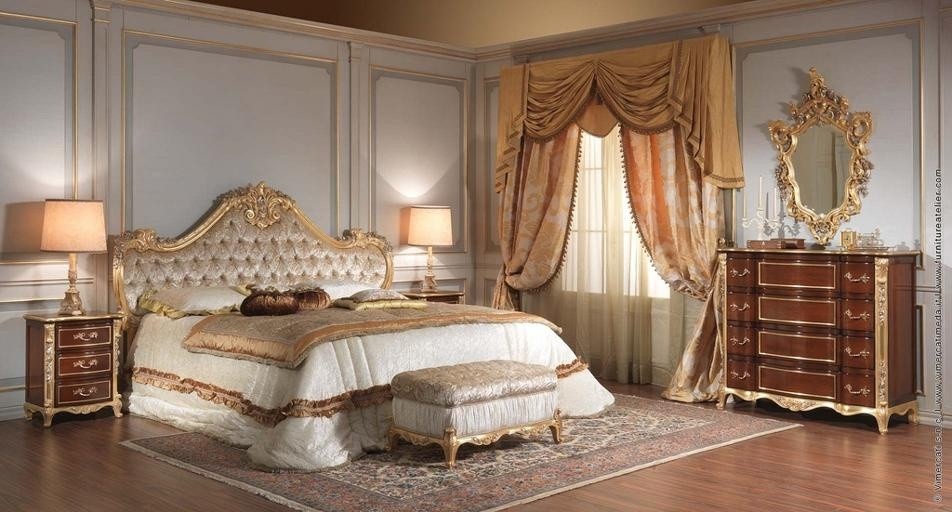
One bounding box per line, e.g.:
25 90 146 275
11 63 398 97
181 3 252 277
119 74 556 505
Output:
715 248 921 432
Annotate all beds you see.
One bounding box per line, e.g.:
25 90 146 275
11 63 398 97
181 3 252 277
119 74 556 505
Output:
113 180 572 470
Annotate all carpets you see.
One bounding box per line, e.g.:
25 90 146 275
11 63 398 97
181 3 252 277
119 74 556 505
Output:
119 388 803 510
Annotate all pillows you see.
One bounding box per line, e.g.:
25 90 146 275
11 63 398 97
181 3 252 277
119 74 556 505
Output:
138 279 428 320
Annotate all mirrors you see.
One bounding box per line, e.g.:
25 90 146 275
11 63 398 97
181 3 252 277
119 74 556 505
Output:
768 67 874 247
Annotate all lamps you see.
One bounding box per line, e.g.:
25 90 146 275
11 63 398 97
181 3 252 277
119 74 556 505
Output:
40 198 110 315
406 205 454 293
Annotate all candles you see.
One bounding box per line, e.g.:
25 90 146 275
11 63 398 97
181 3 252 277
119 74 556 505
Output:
743 175 777 220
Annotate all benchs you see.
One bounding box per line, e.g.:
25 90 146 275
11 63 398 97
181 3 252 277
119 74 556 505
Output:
390 358 563 467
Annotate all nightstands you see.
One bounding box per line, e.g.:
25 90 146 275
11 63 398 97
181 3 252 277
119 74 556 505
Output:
22 313 126 428
